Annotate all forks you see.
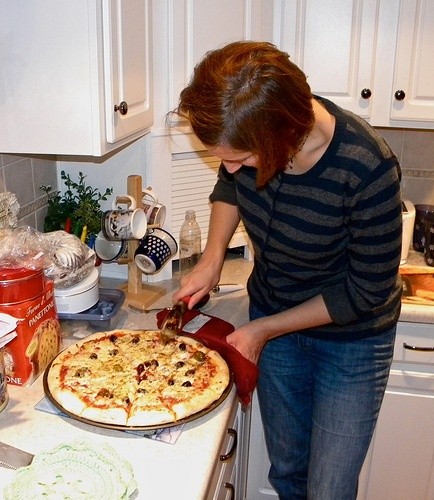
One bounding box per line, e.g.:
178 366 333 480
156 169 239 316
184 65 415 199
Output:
129 305 172 313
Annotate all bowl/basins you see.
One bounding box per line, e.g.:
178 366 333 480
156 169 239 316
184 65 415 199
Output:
8 442 146 500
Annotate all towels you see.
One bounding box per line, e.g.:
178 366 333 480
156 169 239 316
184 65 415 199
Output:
154 305 259 414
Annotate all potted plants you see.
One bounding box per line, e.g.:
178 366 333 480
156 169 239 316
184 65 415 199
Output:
40 170 113 280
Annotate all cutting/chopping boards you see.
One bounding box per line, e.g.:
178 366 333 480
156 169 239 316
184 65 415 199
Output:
42 294 236 428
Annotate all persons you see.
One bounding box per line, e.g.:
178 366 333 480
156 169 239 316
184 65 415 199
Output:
170 39 406 500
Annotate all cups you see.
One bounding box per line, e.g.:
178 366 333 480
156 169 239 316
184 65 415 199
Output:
112 188 166 227
100 195 147 240
95 232 140 263
132 227 178 276
414 199 434 268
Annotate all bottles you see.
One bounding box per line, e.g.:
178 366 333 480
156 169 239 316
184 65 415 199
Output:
179 209 201 276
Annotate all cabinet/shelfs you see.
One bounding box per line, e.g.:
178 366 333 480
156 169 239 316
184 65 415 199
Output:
244 315 434 500
150 0 277 138
277 2 434 132
1 0 155 159
204 392 250 500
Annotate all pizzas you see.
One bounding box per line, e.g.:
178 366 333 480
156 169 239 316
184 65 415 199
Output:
47 328 229 427
